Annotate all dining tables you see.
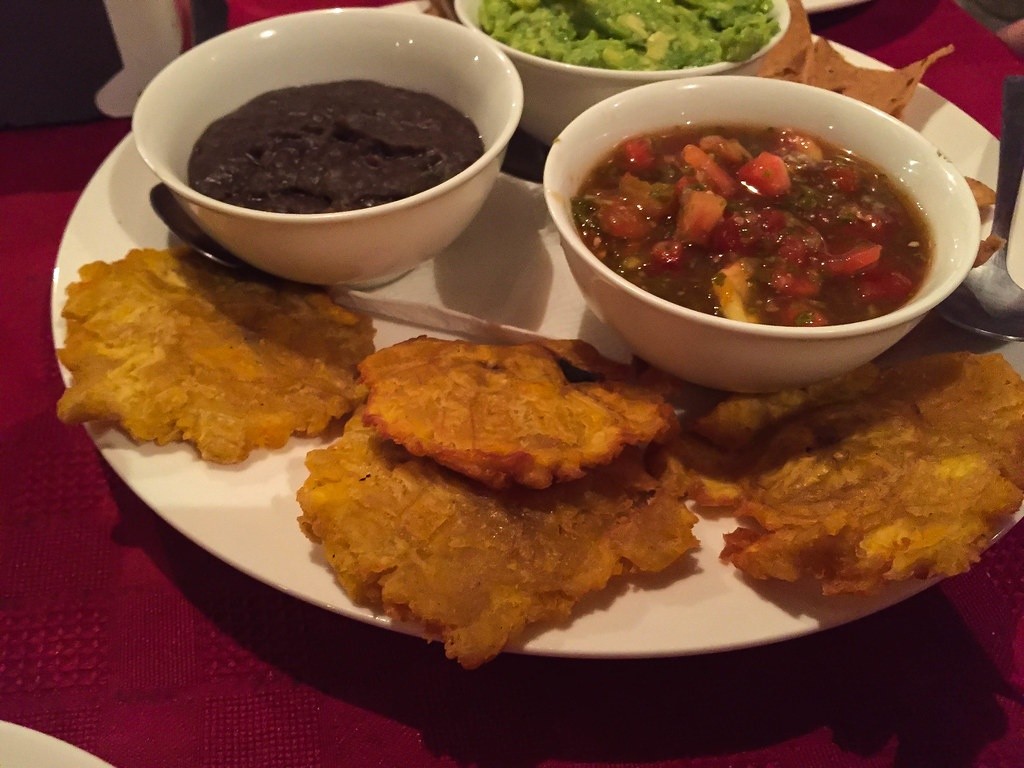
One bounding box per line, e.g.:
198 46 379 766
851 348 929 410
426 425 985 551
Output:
0 0 1024 768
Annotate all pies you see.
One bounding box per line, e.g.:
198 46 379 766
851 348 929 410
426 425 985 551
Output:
55 245 1024 670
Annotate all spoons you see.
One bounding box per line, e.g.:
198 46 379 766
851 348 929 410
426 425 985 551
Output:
148 182 544 347
936 72 1024 340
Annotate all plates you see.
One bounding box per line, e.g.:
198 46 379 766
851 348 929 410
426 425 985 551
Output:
49 0 1024 656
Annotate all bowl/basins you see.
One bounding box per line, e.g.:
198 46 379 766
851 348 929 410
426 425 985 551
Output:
541 77 983 389
132 7 525 292
450 0 791 151
